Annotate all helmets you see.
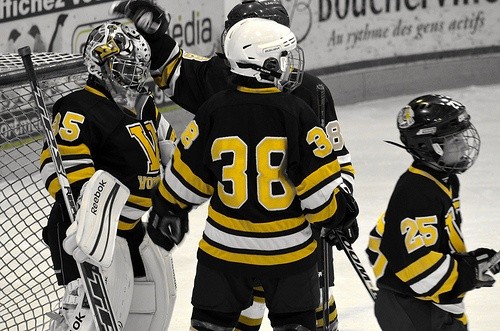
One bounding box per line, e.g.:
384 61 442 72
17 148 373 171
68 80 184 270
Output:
225 0 290 30
83 21 152 80
397 94 471 162
225 18 297 84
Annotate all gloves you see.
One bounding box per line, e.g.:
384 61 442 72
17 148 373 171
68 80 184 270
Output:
469 248 500 289
147 192 189 252
321 220 359 251
110 0 170 41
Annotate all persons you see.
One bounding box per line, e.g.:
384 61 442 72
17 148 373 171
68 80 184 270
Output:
112 0 360 331
366 95 500 331
150 19 359 331
39 20 188 331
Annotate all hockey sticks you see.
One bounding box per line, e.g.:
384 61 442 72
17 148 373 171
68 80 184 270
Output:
18 46 119 331
333 227 379 302
317 83 331 329
